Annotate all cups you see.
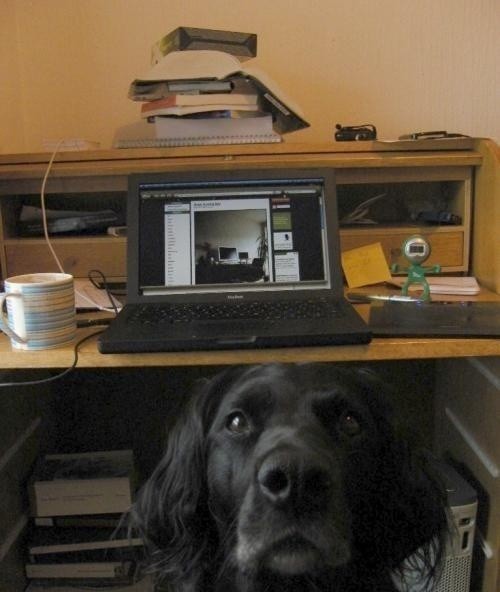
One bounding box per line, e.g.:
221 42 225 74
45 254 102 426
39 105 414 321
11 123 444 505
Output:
0 271 78 351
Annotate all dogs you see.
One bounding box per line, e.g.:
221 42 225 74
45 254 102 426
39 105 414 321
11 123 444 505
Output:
111 361 458 592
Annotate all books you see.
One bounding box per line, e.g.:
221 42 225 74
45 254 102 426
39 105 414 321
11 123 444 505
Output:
385 274 483 296
114 74 311 149
20 447 150 592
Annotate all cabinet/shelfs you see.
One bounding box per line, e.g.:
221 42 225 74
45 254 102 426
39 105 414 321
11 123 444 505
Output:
2 136 484 297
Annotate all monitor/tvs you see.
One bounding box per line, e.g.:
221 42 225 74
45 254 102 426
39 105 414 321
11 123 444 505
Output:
238 252 248 259
218 246 239 262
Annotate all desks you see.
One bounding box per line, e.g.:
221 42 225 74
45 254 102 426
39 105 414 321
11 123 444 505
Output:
1 277 500 456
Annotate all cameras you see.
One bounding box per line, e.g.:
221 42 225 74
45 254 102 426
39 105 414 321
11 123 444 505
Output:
334 125 376 141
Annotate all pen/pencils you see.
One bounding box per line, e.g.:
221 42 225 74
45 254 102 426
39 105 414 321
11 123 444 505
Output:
347 293 413 302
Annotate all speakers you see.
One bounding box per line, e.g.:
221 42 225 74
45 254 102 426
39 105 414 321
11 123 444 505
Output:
430 455 480 592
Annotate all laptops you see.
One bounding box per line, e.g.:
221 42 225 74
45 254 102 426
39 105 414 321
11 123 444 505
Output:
96 166 373 353
